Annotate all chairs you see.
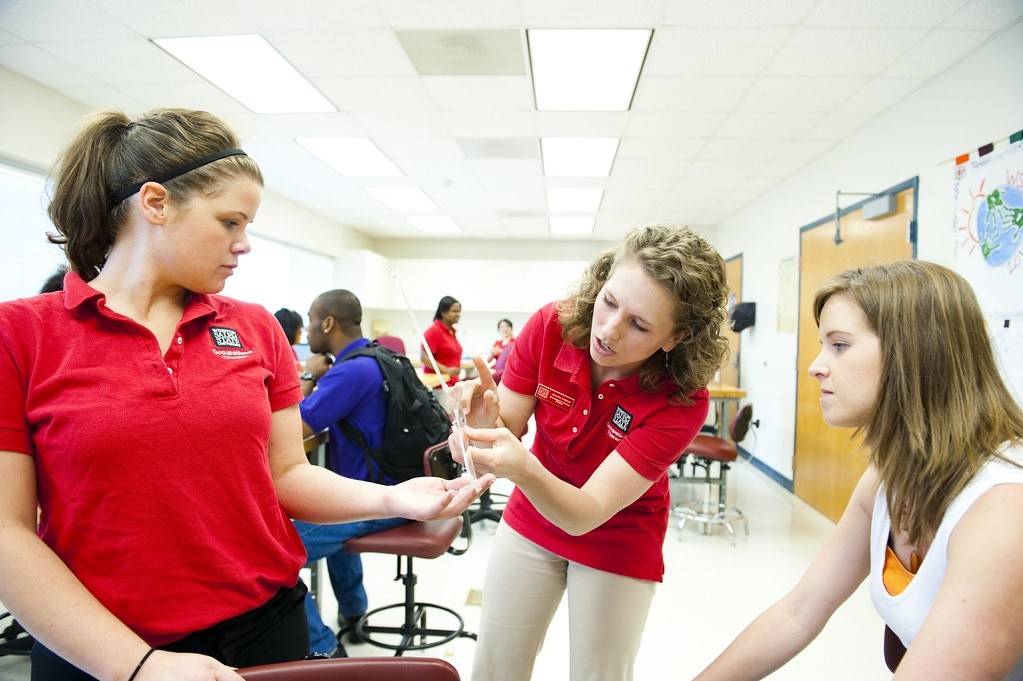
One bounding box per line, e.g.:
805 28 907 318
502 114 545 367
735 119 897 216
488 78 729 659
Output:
345 440 478 658
669 404 760 542
460 488 504 538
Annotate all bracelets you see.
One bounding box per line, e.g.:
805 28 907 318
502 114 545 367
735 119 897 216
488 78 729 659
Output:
128 647 157 681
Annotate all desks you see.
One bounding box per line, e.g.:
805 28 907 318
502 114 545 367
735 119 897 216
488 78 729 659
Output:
304 373 451 453
410 360 474 377
708 380 746 525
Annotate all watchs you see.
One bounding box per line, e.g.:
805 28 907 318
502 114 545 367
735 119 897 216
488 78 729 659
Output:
300 372 317 388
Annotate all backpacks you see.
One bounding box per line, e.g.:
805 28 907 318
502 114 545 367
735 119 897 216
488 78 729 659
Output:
329 338 450 483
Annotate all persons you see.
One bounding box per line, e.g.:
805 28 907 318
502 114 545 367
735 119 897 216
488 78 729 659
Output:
691 259 1022 681
0 107 496 681
421 296 462 413
488 319 516 369
448 224 732 681
292 288 408 659
274 307 304 371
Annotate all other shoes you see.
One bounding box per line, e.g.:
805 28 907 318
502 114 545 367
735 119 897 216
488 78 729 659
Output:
338 605 369 643
309 641 345 658
